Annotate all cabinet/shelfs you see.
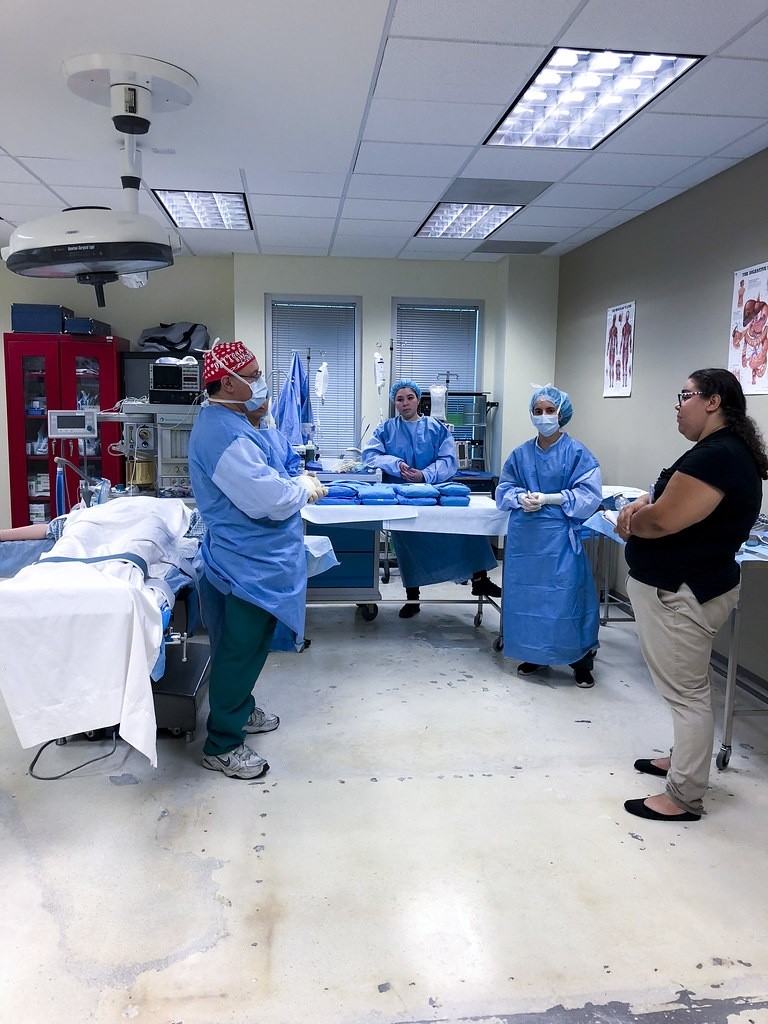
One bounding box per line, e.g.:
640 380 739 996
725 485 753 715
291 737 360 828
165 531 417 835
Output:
2 331 130 529
300 466 383 621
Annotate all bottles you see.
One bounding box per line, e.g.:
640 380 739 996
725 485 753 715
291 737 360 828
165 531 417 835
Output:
612 491 631 512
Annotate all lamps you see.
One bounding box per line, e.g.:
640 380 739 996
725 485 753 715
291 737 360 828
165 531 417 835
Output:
2 55 204 308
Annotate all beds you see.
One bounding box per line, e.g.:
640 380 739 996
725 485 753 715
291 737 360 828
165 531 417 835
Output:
0 499 213 747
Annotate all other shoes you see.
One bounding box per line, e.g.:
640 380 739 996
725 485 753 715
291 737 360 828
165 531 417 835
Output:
634 758 668 776
624 797 701 821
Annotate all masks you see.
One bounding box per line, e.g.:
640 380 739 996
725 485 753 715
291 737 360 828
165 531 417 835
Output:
234 375 268 412
530 412 562 437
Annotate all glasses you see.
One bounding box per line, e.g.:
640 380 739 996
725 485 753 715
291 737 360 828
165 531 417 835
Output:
678 391 708 408
237 371 263 381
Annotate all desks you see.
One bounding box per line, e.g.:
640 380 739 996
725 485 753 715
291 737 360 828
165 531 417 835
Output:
583 511 768 768
295 492 509 653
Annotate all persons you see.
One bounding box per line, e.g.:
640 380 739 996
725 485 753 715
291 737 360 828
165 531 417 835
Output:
497 385 602 689
614 368 768 821
187 342 329 781
0 517 68 543
362 377 502 618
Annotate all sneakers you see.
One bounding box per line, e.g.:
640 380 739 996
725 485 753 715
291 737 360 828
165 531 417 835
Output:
471 578 501 598
573 669 594 688
242 706 280 734
399 602 420 618
202 741 270 780
517 662 549 675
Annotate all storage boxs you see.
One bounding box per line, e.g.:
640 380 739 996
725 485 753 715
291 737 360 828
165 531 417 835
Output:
9 302 113 336
